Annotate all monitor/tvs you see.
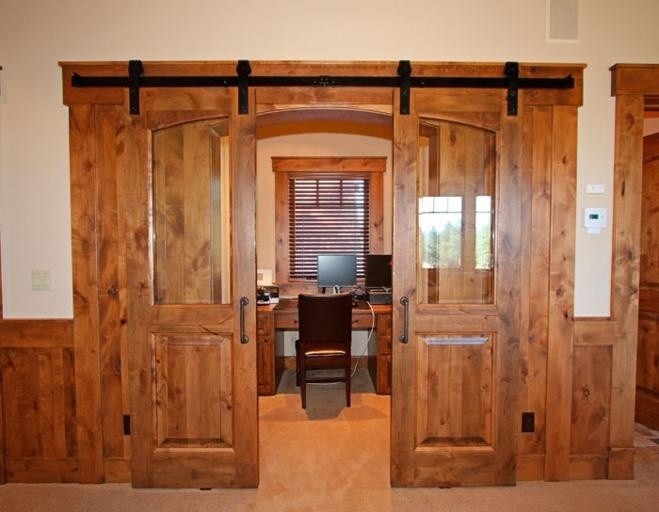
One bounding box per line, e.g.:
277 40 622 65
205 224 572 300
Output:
317 255 357 294
366 256 393 289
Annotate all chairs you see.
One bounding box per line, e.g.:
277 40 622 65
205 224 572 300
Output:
295 292 352 408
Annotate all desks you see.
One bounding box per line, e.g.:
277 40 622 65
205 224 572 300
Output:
256 293 393 398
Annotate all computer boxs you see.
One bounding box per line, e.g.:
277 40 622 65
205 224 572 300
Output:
367 290 392 305
